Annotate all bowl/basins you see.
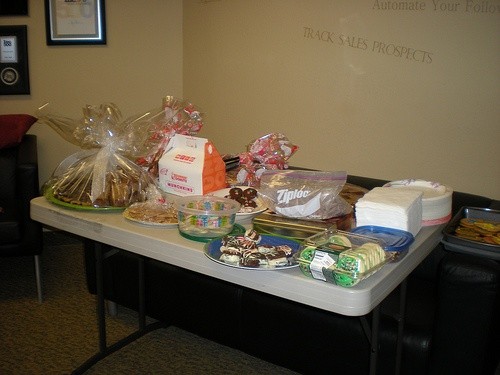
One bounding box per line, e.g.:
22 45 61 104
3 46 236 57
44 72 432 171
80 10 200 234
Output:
206 188 269 222
175 195 240 237
0 114 38 143
351 225 414 262
326 213 353 231
294 228 394 287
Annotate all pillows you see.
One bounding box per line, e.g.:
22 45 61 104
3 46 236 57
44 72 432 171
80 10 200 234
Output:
0 114 39 149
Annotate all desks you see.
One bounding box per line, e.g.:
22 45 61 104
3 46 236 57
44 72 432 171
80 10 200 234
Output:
29 195 449 375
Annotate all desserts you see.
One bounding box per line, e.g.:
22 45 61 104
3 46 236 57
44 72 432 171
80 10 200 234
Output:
219 229 294 268
223 188 257 209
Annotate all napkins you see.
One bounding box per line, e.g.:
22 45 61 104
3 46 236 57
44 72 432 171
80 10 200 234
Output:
355 187 422 237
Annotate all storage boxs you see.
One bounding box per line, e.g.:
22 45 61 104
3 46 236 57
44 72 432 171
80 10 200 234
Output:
158 134 226 196
252 211 351 243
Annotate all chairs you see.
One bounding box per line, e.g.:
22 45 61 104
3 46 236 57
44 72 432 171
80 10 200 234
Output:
0 135 43 305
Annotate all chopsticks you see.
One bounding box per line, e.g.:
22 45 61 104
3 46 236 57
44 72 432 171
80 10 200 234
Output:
252 218 326 234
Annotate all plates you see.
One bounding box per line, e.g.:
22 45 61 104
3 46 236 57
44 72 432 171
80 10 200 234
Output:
340 182 369 204
205 235 304 270
123 203 178 228
253 214 336 243
45 188 125 213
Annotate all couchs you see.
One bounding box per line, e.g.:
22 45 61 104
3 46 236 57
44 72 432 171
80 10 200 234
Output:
85 166 500 375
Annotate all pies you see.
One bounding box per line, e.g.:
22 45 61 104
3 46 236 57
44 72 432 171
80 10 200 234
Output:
455 218 500 245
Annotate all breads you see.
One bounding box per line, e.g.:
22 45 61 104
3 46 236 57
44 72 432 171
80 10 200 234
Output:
297 234 386 288
57 169 150 209
